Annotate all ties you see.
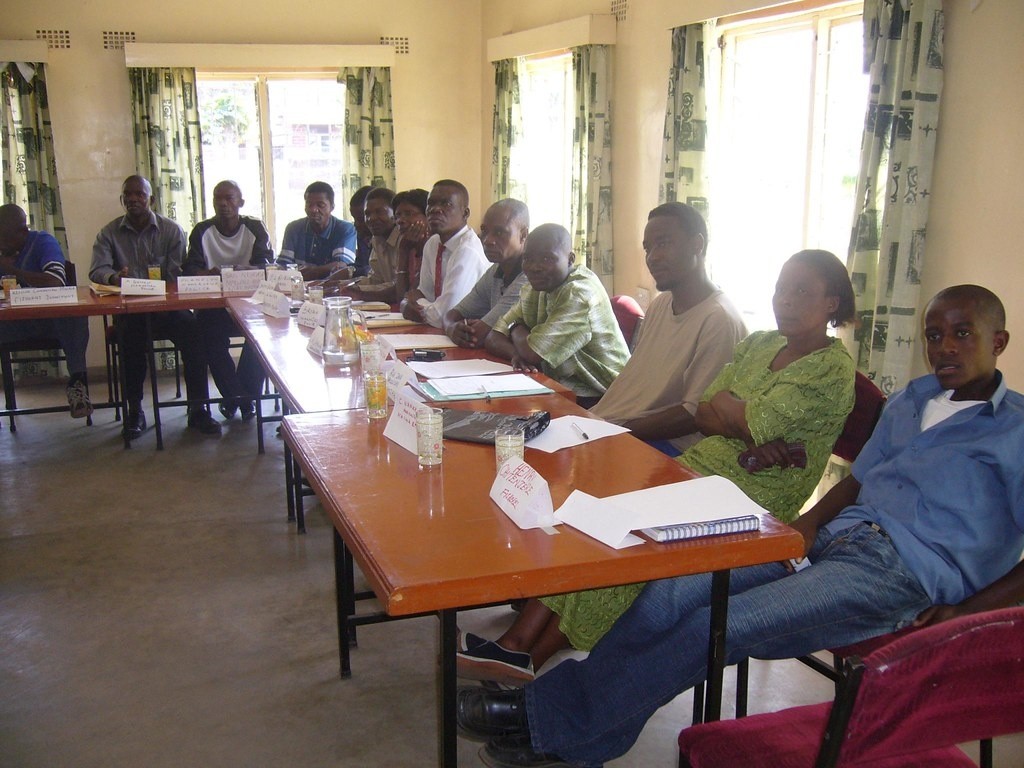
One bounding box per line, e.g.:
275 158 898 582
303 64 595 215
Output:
433 244 447 296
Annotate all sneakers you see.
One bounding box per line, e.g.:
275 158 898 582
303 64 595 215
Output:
457 632 536 691
188 407 221 434
218 399 239 419
120 410 147 439
65 380 94 418
239 398 258 420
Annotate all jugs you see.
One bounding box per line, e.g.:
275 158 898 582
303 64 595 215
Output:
322 296 368 366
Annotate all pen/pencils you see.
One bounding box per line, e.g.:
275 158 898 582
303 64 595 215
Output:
365 314 389 321
333 279 361 292
570 418 589 440
481 385 491 400
464 316 469 341
265 257 271 265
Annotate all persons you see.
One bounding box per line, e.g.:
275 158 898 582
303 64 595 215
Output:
184 180 633 423
456 283 1024 768
0 204 91 419
89 174 223 438
509 199 749 615
457 248 855 690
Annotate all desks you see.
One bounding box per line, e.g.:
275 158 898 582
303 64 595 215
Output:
0 262 806 768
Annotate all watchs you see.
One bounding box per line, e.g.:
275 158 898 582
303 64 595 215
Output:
508 320 523 333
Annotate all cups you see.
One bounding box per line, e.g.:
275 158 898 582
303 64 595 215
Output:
220 264 233 274
265 263 279 280
415 407 444 471
308 286 323 305
286 263 299 271
364 369 388 422
2 275 17 300
494 428 525 476
147 264 161 280
359 340 381 382
290 276 305 302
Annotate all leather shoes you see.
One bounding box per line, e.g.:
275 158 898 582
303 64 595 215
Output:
456 685 605 768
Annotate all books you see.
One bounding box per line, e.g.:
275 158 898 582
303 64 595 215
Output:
639 474 761 542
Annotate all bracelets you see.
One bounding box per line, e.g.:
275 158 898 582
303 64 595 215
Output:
395 270 407 275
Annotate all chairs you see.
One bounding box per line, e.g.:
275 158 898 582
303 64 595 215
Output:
609 295 645 355
0 260 93 432
678 605 1024 768
102 315 180 421
693 371 887 724
736 556 1024 768
